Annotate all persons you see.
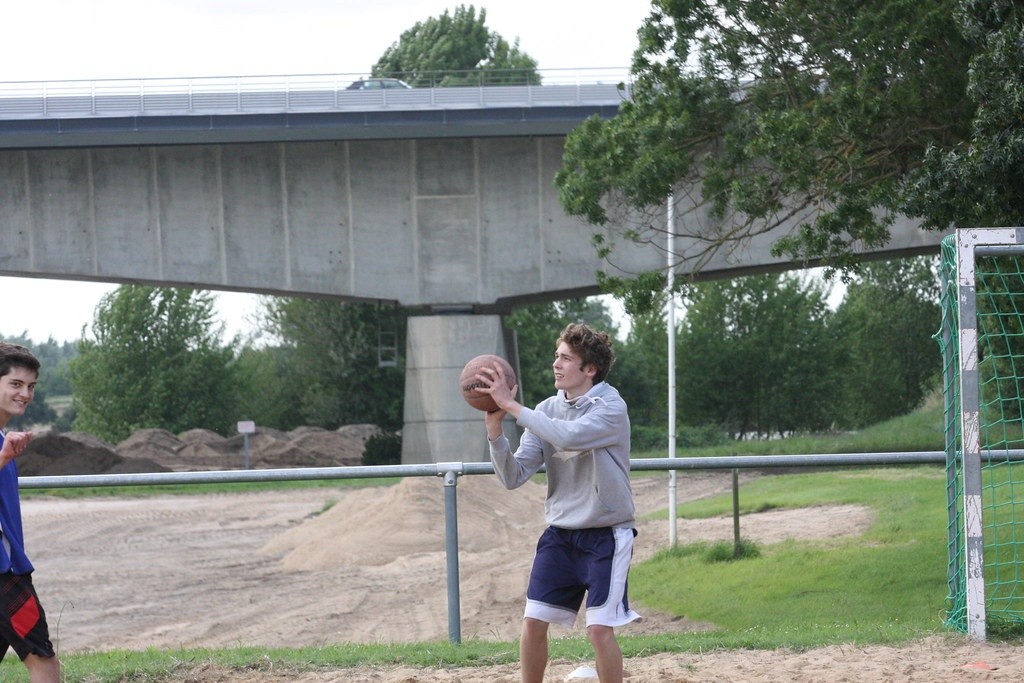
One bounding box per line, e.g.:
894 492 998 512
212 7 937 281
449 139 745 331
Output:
473 321 643 683
0 343 61 683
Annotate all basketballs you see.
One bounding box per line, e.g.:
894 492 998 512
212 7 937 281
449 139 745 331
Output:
458 354 516 412
